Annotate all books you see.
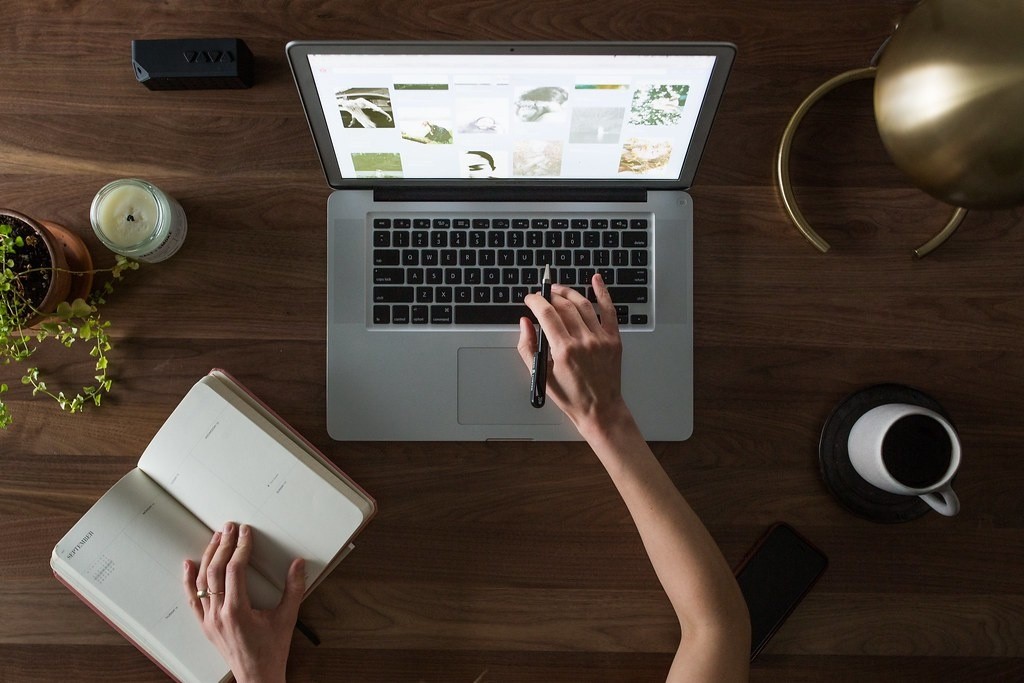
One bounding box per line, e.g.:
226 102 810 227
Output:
49 368 378 683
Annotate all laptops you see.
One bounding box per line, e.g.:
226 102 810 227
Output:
285 40 739 442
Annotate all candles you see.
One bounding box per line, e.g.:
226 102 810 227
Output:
91 179 187 264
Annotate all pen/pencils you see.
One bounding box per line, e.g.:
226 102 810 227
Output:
530 264 552 409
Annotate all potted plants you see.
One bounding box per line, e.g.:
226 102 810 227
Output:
1 207 141 430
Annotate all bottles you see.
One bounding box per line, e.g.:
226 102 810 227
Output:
87 180 188 263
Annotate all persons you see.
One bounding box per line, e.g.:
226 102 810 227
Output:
183 274 751 683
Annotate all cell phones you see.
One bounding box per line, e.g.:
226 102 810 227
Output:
733 521 829 663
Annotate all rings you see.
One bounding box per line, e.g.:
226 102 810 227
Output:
207 588 225 597
197 591 207 598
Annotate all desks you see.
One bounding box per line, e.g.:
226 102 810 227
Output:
0 0 1024 683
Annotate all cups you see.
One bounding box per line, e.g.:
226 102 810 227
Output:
848 405 962 515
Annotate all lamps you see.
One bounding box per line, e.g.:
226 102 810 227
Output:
778 0 1024 257
